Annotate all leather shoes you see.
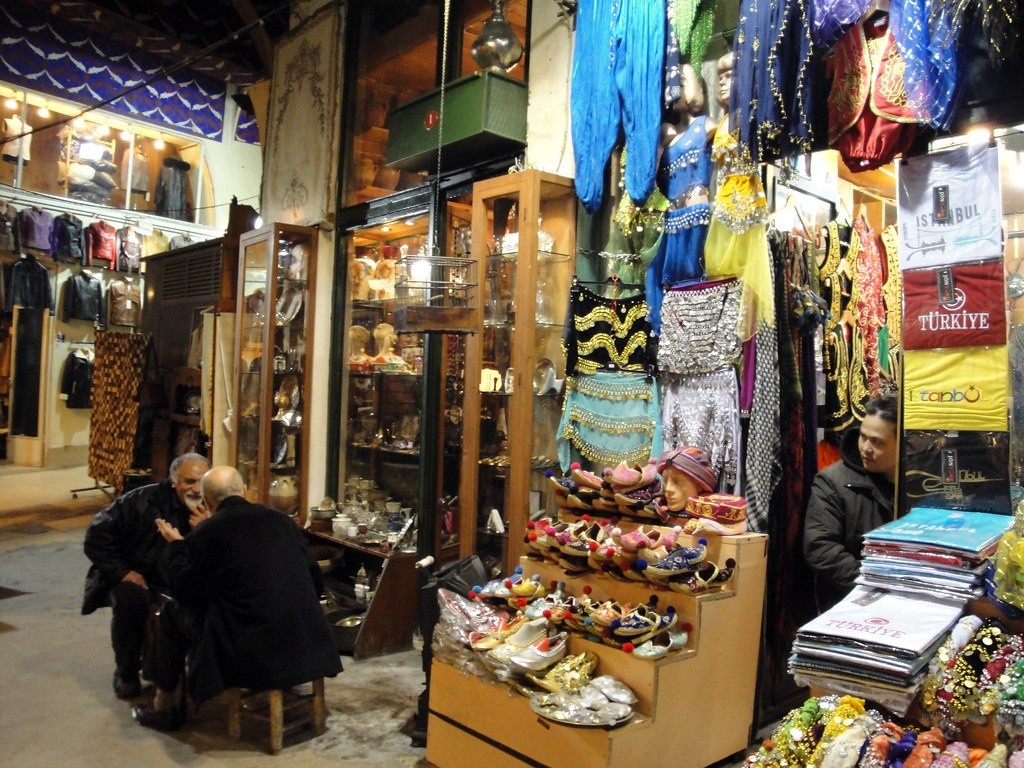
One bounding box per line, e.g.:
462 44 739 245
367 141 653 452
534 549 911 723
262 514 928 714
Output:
132 701 183 732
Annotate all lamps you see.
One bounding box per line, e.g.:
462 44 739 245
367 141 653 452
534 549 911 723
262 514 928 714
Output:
471 0 524 75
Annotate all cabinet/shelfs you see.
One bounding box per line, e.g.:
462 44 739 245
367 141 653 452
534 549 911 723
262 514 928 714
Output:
229 221 318 525
300 513 420 659
421 512 768 768
339 200 473 468
59 128 117 204
348 124 398 202
458 169 579 580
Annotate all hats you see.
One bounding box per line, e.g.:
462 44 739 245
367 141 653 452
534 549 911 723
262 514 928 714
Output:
660 447 719 494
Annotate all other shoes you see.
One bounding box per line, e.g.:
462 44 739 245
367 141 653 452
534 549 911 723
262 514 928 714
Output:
464 462 737 695
112 668 141 697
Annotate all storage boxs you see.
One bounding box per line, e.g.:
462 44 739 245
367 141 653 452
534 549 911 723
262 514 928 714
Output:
384 68 527 174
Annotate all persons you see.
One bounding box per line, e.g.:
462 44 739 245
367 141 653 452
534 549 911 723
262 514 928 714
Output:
657 448 715 513
132 466 344 733
82 453 215 701
801 394 907 611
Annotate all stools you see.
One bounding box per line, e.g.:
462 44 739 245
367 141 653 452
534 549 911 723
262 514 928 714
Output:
227 678 328 752
